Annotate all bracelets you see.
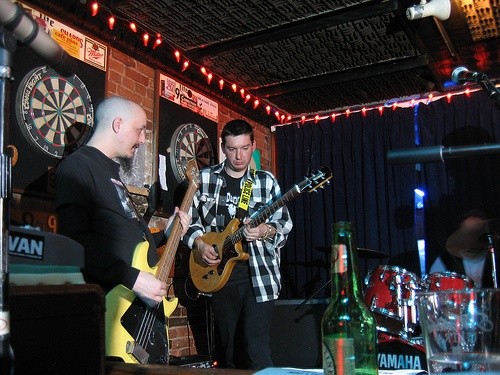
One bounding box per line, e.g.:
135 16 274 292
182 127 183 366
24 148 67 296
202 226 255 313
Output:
263 225 271 240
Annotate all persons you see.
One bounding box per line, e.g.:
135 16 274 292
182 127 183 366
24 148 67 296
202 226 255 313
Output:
434 216 500 289
52 96 192 365
182 120 293 370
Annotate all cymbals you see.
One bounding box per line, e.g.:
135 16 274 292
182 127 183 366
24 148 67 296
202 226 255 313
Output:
316 244 388 260
446 218 500 260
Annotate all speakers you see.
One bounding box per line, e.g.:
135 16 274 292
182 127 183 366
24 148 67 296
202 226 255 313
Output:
269 298 329 369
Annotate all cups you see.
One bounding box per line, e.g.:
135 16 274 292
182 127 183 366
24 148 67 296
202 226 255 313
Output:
415 287 500 375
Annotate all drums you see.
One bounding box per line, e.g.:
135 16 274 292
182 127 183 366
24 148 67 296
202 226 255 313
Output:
364 265 423 328
374 322 428 375
421 271 475 345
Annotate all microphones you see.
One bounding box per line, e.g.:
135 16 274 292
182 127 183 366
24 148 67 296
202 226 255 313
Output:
451 67 487 83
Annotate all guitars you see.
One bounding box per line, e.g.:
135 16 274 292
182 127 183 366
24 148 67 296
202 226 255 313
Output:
189 167 333 294
103 173 200 362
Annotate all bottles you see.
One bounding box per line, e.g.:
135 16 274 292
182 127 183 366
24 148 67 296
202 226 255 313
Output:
320 221 378 375
